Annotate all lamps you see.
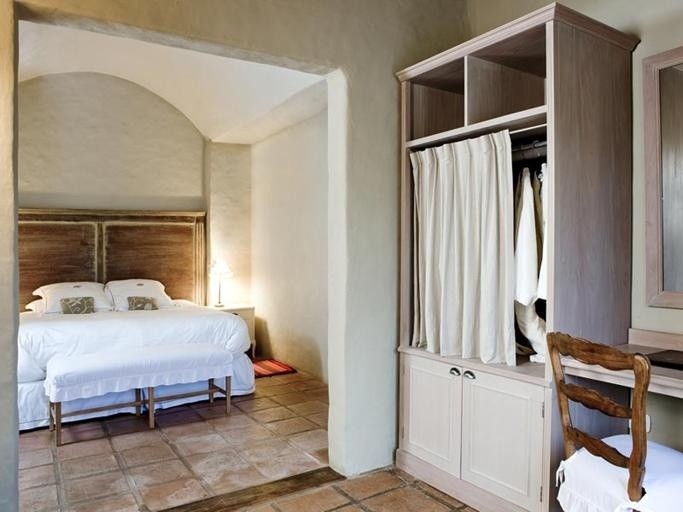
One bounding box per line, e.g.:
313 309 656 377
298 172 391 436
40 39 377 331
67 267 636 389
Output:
205 260 236 308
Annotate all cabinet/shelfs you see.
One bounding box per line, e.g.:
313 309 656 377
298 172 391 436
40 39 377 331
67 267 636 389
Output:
378 0 645 512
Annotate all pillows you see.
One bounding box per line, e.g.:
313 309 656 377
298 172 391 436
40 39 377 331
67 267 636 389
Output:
19 275 183 315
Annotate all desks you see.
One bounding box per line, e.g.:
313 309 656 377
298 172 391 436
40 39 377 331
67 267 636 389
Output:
522 324 683 402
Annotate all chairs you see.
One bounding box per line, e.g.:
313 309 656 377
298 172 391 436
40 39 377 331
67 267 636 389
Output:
538 330 683 512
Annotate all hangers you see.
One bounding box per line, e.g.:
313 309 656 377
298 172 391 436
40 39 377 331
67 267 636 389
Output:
514 143 528 184
529 136 547 180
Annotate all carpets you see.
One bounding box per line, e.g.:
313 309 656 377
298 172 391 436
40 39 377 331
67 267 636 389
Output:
245 356 298 381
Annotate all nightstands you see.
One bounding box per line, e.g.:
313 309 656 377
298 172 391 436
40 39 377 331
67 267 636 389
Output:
204 301 257 361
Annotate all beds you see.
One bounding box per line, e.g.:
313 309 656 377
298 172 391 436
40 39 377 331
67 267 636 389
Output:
8 203 259 435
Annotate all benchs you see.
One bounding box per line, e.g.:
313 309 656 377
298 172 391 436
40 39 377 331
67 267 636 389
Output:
41 340 234 449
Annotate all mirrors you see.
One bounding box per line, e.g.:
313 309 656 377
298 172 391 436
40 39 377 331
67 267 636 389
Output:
639 44 682 312
